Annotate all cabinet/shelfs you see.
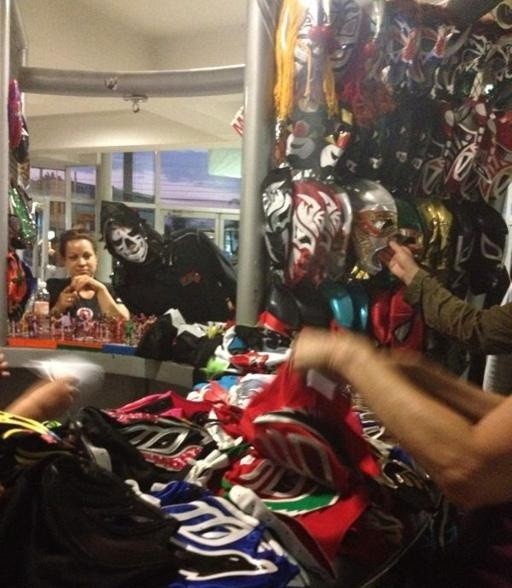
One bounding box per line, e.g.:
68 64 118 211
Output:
0 348 193 408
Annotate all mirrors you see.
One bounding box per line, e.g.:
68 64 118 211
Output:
17 148 241 325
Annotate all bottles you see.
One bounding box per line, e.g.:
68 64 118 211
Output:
32 280 51 339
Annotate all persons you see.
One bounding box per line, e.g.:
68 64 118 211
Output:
102 212 238 324
389 240 512 356
291 326 512 584
1 351 78 426
47 230 124 321
